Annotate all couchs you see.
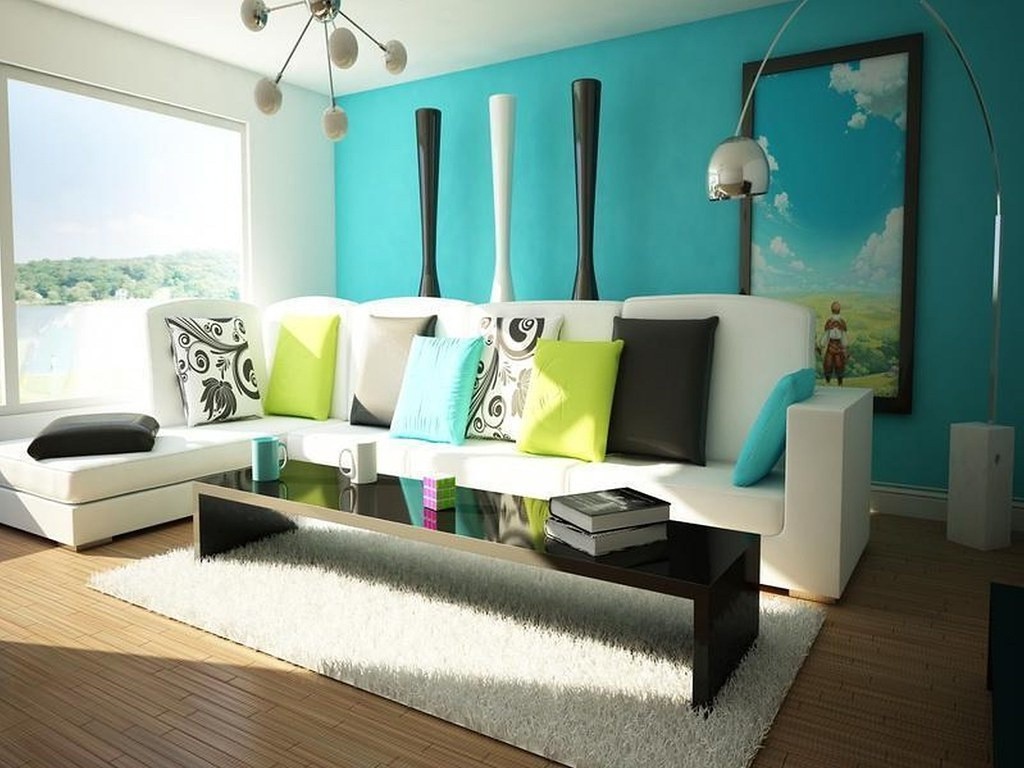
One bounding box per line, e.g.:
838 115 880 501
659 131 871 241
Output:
0 296 874 604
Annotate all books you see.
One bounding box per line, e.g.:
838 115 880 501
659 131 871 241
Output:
543 487 671 557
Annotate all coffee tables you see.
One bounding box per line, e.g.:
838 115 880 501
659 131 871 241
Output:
192 457 759 718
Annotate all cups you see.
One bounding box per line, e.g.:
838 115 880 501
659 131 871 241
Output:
251 436 287 482
252 480 288 500
338 440 377 484
338 484 377 518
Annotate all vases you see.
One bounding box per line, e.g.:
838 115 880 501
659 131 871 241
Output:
486 95 516 304
571 77 599 301
415 108 442 297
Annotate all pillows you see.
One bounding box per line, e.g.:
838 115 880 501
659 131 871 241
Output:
349 316 436 427
164 316 263 426
464 316 564 443
520 337 625 460
608 312 721 465
386 337 485 443
732 365 816 486
26 414 159 459
264 315 339 418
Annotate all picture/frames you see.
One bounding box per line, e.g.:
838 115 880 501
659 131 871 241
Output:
736 32 922 411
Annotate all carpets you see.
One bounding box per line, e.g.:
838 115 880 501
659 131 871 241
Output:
88 516 827 767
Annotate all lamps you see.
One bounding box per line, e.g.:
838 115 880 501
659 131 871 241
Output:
707 1 1014 551
242 0 408 140
